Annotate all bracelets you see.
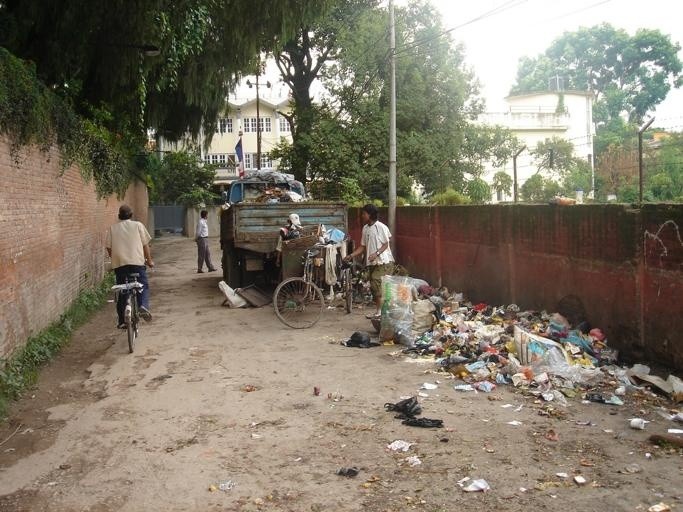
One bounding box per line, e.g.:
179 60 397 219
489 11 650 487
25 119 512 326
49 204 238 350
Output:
350 253 353 258
376 250 380 256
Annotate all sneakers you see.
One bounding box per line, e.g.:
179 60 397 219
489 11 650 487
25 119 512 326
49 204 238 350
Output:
117 323 127 329
366 313 381 319
196 267 218 273
139 305 152 322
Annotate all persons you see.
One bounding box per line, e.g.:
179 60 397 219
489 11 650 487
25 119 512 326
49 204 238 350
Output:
341 203 396 321
194 211 218 274
275 214 301 268
104 203 156 329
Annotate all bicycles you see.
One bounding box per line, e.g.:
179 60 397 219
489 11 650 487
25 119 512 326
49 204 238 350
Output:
120 259 153 353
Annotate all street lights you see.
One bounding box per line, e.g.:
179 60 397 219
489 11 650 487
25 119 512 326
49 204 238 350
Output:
246 75 271 169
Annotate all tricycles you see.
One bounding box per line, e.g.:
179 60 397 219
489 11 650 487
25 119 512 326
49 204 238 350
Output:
272 233 356 329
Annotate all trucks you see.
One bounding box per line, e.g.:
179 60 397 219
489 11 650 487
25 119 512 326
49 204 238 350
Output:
220 180 349 288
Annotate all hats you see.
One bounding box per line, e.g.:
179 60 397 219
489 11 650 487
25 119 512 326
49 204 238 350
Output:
118 205 134 215
288 213 302 225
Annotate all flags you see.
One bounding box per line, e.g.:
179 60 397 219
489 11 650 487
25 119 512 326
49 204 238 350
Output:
234 137 245 177
